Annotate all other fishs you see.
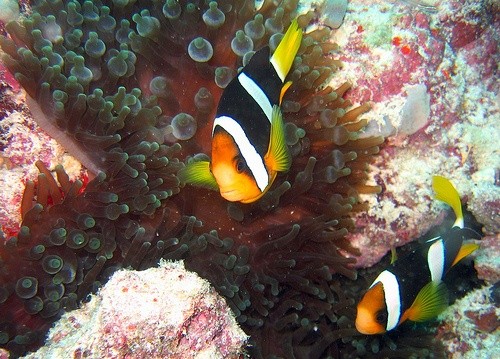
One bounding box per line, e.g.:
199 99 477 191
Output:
177 20 303 205
354 175 478 335
